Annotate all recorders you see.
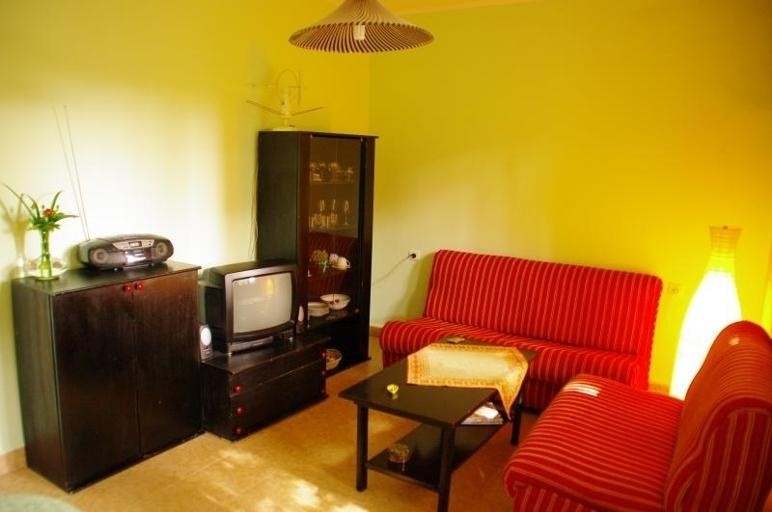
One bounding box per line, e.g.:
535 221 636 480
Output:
76 234 173 272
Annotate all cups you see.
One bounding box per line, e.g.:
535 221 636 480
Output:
329 253 351 270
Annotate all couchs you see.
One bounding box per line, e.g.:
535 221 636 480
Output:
378 248 665 415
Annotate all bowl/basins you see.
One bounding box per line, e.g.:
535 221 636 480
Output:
320 294 351 311
308 303 329 317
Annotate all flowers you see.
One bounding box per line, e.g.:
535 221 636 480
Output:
3 180 81 278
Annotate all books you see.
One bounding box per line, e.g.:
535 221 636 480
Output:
459 401 504 425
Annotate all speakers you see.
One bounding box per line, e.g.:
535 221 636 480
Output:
295 305 307 334
199 323 216 363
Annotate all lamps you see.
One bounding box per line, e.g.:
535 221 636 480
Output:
288 1 435 55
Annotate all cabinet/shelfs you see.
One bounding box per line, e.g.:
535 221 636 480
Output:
201 332 332 442
10 259 204 494
255 130 380 380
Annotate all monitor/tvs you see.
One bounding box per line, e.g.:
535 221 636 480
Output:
199 258 301 356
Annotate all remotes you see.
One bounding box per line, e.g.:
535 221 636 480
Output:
475 406 499 419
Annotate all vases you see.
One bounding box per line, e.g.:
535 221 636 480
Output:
24 243 70 280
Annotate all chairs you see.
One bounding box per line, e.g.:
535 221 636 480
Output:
500 318 772 511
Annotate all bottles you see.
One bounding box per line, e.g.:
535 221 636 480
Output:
317 198 338 230
